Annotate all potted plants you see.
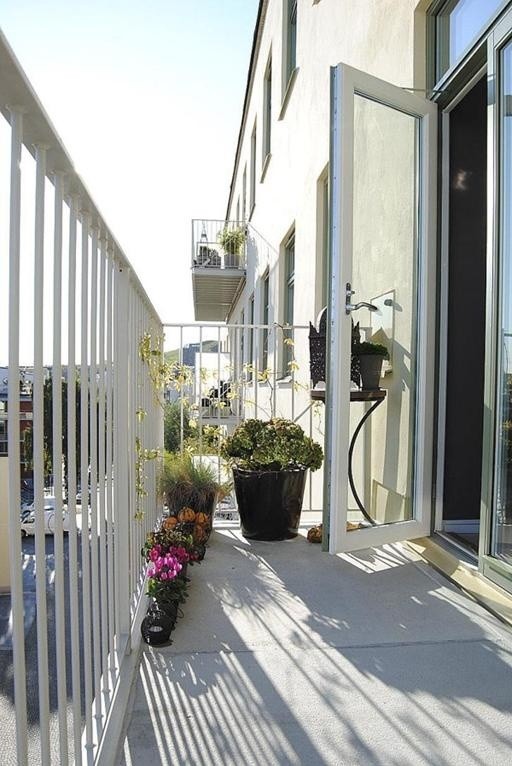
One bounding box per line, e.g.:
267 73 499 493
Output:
218 226 245 269
158 417 324 541
351 335 388 391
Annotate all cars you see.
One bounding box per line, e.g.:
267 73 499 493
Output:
76 493 91 505
20 496 69 537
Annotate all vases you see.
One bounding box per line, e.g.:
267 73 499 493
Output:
141 603 177 645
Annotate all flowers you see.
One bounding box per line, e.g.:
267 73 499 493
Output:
142 531 194 602
